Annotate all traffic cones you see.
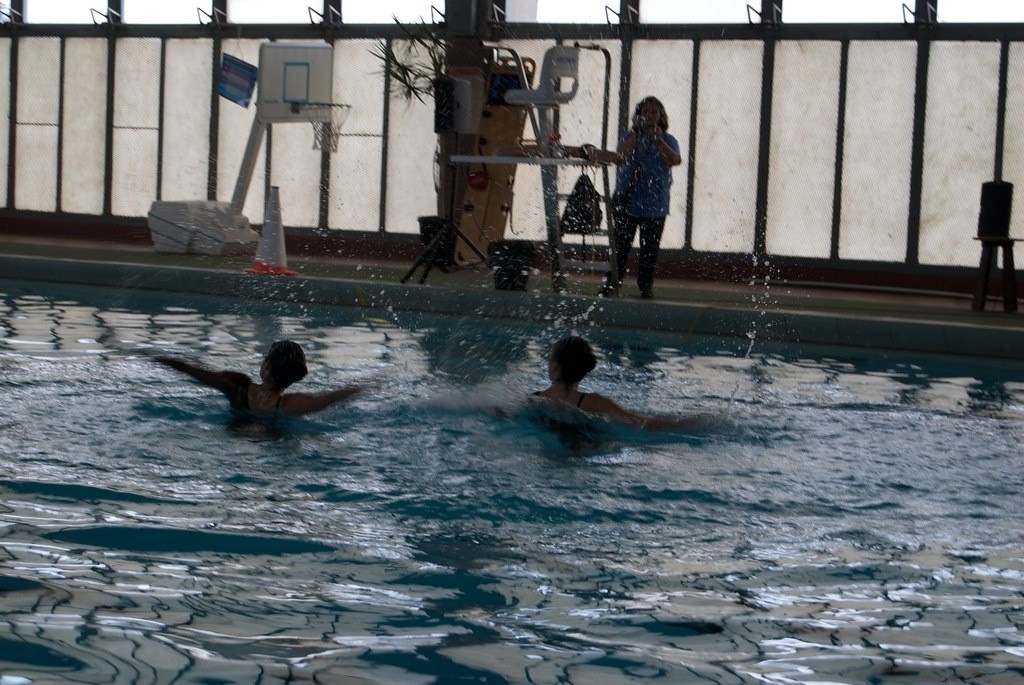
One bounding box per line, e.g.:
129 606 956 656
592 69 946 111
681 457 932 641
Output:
244 185 300 278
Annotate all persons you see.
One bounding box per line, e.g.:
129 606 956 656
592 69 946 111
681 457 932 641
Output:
532 336 715 439
152 341 377 439
596 96 681 300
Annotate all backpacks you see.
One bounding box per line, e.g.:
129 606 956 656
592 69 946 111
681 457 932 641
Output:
560 174 603 232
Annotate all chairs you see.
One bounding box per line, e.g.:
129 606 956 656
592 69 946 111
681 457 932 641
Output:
504 45 580 154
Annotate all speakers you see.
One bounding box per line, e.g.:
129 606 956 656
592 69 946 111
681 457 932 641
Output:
449 73 484 135
445 0 506 42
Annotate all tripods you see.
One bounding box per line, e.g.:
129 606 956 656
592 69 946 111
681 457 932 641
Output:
401 133 488 284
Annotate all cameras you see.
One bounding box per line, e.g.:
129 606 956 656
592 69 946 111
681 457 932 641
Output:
638 117 656 130
